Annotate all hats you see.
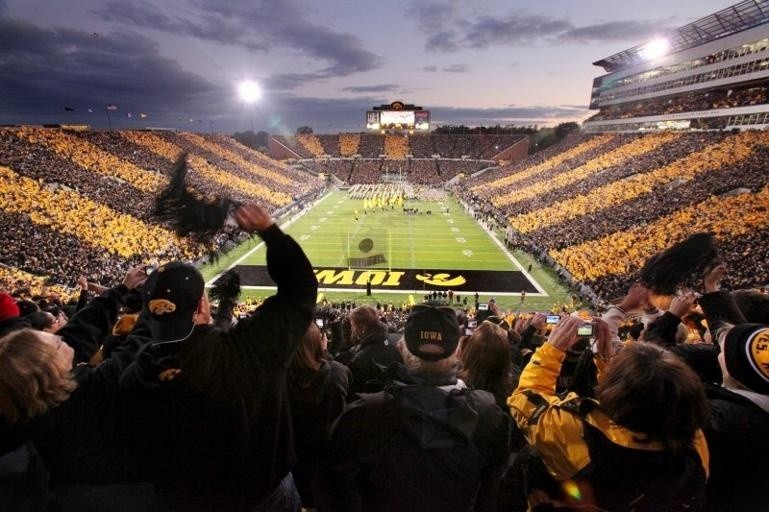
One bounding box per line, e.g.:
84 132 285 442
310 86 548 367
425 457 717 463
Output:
724 322 769 395
404 300 461 360
143 261 205 347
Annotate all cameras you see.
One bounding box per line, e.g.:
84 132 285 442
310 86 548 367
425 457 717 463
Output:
545 314 560 324
145 266 155 275
577 322 595 336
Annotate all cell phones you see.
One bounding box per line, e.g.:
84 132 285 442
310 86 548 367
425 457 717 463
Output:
315 317 325 332
